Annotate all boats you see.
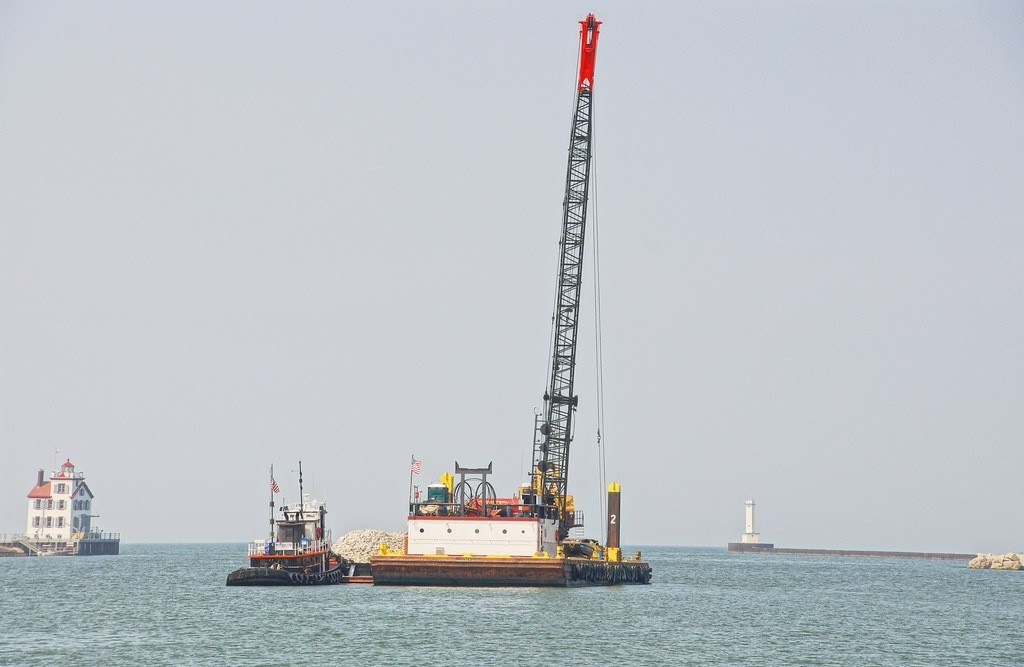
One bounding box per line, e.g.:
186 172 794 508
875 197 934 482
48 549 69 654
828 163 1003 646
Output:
357 15 658 589
225 459 349 587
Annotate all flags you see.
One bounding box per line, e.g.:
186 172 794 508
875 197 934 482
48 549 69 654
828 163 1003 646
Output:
271 477 280 493
412 458 421 475
